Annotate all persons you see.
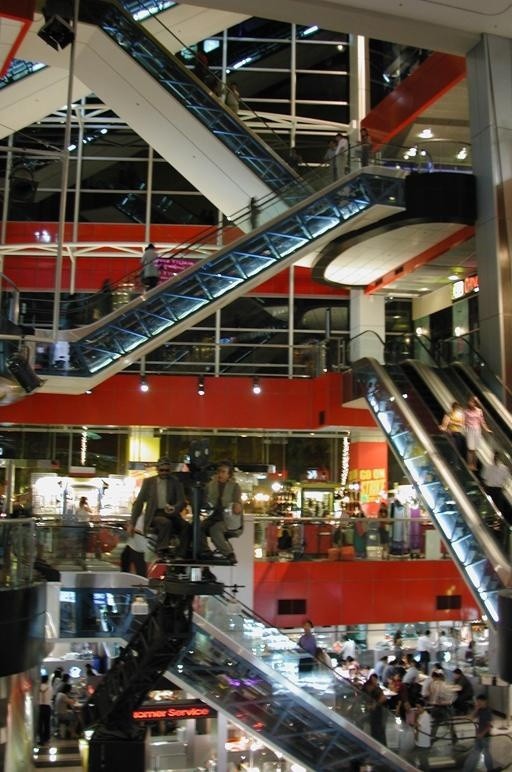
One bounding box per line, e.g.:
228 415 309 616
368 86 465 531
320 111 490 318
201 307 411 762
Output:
296 620 495 772
37 663 96 745
442 397 508 509
193 50 240 115
65 243 160 333
324 129 373 183
249 196 263 230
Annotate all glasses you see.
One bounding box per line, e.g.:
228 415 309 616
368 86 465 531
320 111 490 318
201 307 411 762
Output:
159 467 170 472
